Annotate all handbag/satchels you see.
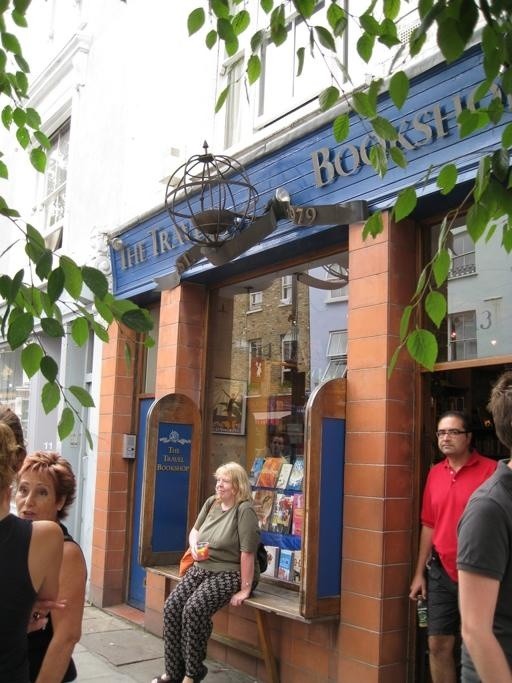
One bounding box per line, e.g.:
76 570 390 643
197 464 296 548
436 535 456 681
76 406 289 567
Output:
178 544 195 578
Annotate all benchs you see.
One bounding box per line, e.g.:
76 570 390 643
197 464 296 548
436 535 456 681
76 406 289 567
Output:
147 564 340 683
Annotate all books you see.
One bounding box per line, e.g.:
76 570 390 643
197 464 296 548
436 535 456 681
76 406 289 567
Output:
249 456 304 584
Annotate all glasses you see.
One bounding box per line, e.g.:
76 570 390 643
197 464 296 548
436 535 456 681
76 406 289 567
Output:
435 429 471 438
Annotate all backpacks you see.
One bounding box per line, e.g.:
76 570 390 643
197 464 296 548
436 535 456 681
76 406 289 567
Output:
256 542 268 574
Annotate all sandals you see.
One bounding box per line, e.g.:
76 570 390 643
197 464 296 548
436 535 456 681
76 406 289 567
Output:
180 662 208 683
149 671 181 683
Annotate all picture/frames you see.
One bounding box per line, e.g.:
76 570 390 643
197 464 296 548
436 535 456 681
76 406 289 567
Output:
209 376 248 436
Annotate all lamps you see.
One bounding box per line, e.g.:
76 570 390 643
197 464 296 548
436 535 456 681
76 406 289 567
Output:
268 187 290 207
110 238 122 250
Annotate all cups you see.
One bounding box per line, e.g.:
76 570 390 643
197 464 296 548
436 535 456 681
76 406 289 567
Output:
191 542 209 561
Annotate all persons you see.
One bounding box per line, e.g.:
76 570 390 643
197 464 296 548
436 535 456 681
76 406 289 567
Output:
150 461 262 683
408 409 500 683
267 433 286 459
0 404 71 683
455 370 511 683
13 451 88 683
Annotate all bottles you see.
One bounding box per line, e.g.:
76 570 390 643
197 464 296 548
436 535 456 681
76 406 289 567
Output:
417 594 429 628
